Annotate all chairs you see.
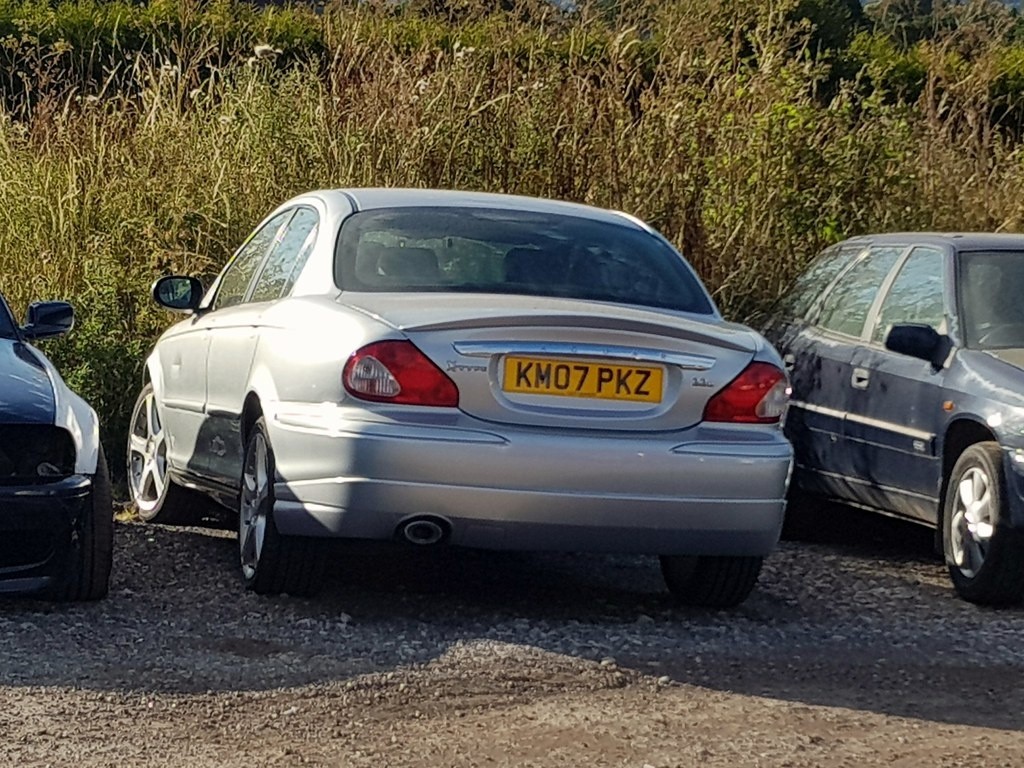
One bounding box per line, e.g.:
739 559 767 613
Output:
504 248 551 281
356 241 386 286
376 247 438 277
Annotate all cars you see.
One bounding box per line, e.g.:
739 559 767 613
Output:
0 277 114 610
126 188 794 610
764 232 1024 609
